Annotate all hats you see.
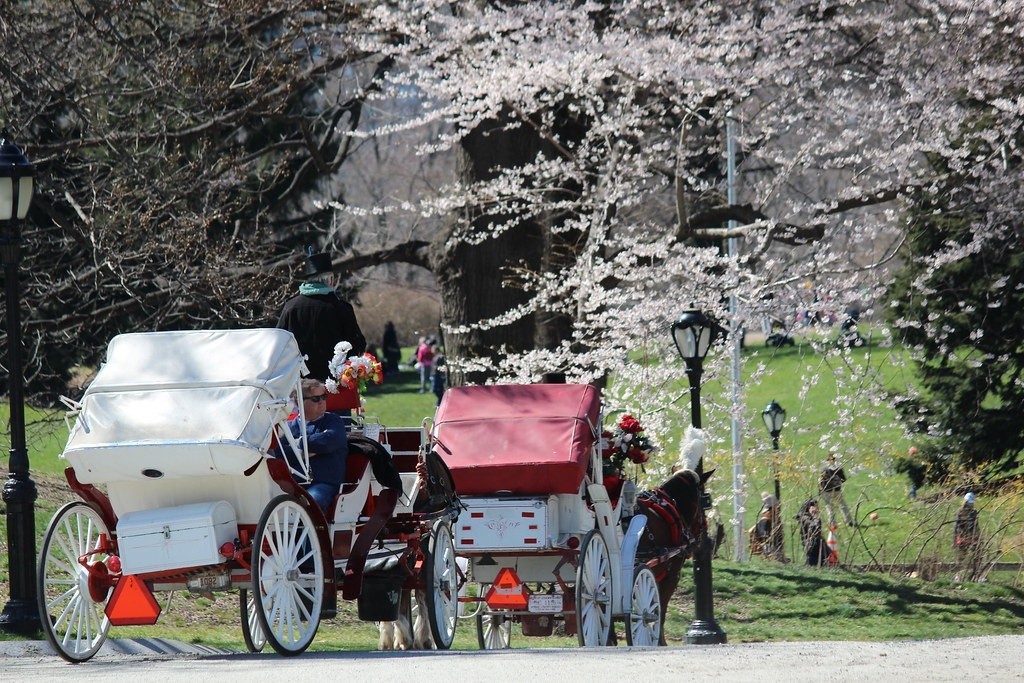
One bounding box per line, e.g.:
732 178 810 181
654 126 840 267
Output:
964 492 974 504
418 336 425 345
305 253 332 283
761 492 779 514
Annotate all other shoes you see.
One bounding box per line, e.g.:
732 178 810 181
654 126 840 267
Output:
416 389 425 394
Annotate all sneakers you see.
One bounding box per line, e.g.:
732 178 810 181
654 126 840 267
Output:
971 575 987 582
955 576 962 589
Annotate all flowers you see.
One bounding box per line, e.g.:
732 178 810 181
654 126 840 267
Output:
325 340 384 424
600 411 660 476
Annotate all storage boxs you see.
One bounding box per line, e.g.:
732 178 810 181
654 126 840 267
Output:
115 500 239 576
454 495 560 551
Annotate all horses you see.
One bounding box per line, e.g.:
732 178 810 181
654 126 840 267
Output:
377 424 454 650
605 458 717 646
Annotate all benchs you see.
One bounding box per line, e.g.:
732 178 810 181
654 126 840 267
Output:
329 450 376 521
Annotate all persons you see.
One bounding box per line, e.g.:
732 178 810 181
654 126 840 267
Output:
792 497 833 565
757 491 785 563
408 353 418 366
418 336 433 394
366 321 401 375
276 246 366 432
951 492 989 583
429 345 446 406
274 379 347 574
909 447 926 497
818 454 854 528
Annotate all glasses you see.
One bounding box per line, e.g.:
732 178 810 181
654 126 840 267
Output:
831 458 835 460
305 394 326 402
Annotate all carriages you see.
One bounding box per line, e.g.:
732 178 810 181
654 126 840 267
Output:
35 328 439 664
417 383 718 651
